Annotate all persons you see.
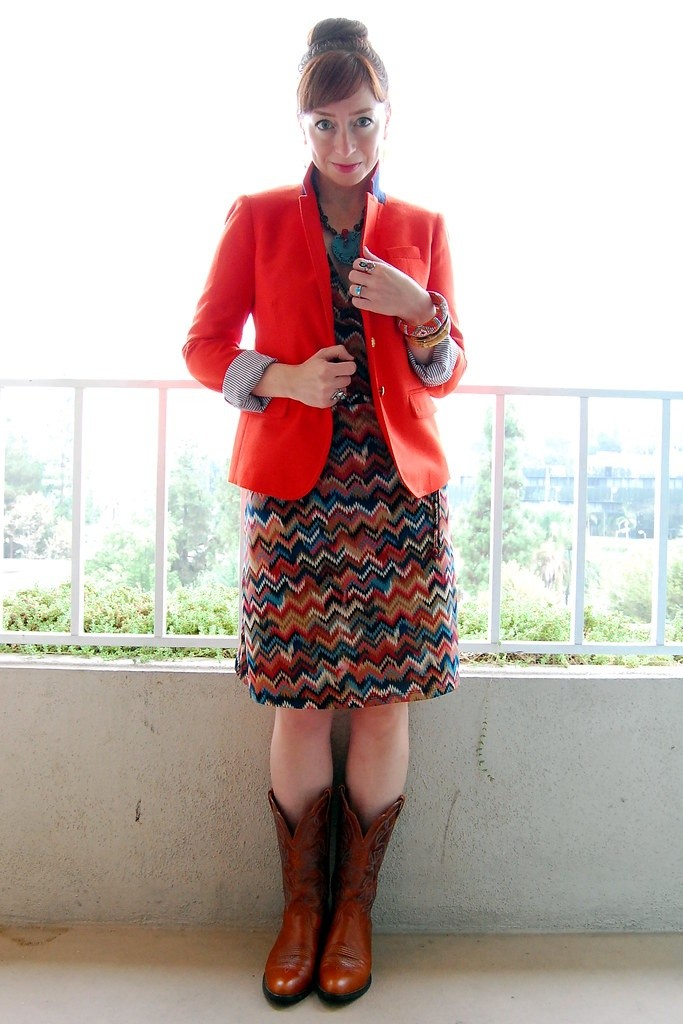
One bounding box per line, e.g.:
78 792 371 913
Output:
181 17 468 1005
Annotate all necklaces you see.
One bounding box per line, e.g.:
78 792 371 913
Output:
317 203 364 267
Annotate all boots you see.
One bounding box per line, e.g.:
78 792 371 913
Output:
263 786 335 1001
316 782 405 1001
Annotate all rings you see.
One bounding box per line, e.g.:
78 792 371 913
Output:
358 260 376 272
355 286 362 296
332 389 346 400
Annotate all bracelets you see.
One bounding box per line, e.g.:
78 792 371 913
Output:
396 291 450 349
